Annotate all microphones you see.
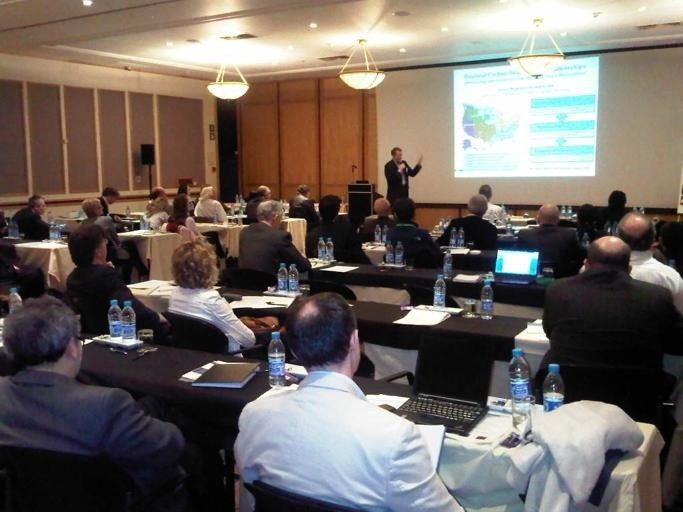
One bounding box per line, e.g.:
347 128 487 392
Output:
400 160 406 172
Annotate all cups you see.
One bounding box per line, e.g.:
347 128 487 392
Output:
510 394 536 430
462 298 479 319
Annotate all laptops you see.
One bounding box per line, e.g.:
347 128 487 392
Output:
482 248 541 285
392 334 496 437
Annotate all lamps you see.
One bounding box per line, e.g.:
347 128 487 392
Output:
506 16 567 79
336 38 386 91
206 62 249 100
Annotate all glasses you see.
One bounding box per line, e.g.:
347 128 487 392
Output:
75 334 86 347
101 238 108 245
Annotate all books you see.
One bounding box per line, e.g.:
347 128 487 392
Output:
192 363 261 388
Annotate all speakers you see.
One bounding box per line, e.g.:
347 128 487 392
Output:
140 143 154 165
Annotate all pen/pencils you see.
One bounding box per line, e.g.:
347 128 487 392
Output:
109 348 128 355
265 302 288 306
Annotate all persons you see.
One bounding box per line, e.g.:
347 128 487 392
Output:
542 236 682 424
384 147 423 203
0 185 321 356
309 184 682 313
1 294 186 511
233 292 465 511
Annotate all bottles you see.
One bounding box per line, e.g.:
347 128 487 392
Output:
383 241 394 264
288 264 300 292
442 249 454 279
479 277 494 320
317 238 325 258
507 346 533 411
541 363 566 414
394 242 404 264
105 299 138 339
266 331 285 389
324 238 334 260
7 286 23 318
230 194 246 227
124 207 150 231
278 263 288 291
46 210 61 240
433 274 447 306
372 199 649 251
7 220 19 238
